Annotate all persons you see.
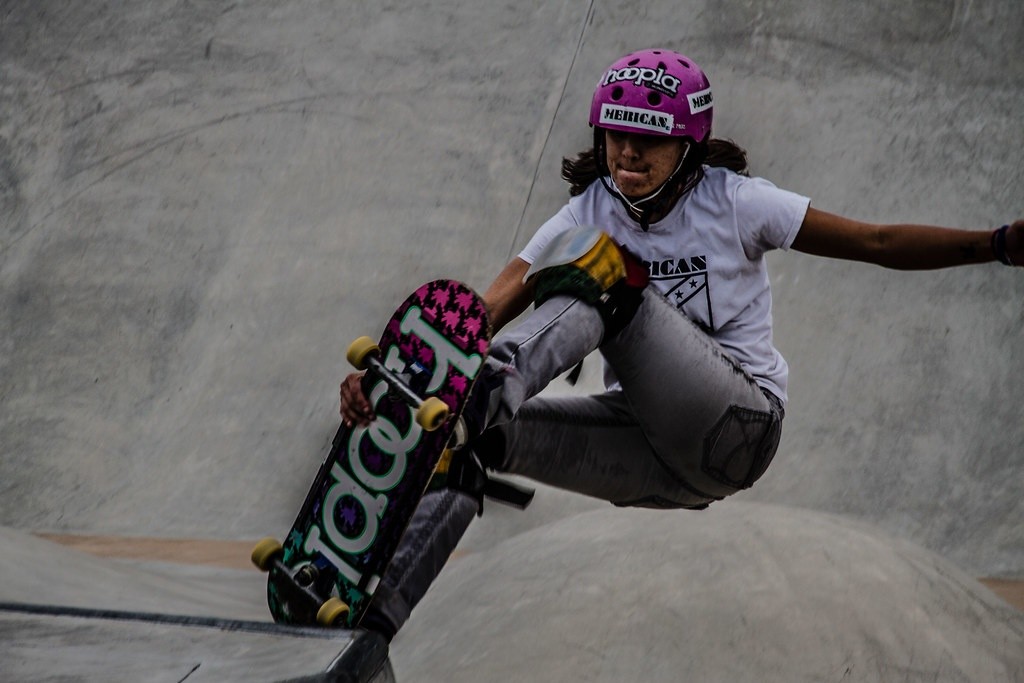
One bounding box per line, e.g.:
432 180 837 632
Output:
337 48 1024 645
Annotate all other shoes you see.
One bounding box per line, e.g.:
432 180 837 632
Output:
445 384 489 451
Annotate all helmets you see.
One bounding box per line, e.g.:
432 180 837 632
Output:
590 48 713 142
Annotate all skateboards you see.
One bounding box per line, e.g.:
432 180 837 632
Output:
249 277 494 630
